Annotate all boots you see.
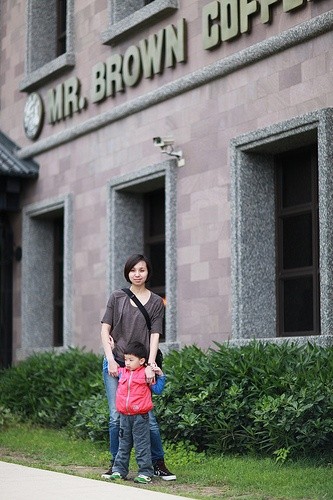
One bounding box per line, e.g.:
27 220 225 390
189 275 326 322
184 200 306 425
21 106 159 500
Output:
101 464 113 478
152 458 176 480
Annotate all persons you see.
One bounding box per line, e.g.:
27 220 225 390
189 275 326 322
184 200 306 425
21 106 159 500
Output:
98 335 166 484
100 253 176 481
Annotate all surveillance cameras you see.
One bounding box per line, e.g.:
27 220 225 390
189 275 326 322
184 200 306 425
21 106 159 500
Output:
152 137 174 146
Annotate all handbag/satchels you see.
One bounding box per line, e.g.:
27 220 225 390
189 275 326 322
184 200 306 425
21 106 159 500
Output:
156 349 164 370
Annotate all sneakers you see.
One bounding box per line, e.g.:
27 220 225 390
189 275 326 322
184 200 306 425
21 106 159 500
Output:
109 472 123 478
134 474 152 483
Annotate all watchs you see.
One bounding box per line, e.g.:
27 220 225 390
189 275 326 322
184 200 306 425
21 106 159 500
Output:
147 363 156 367
